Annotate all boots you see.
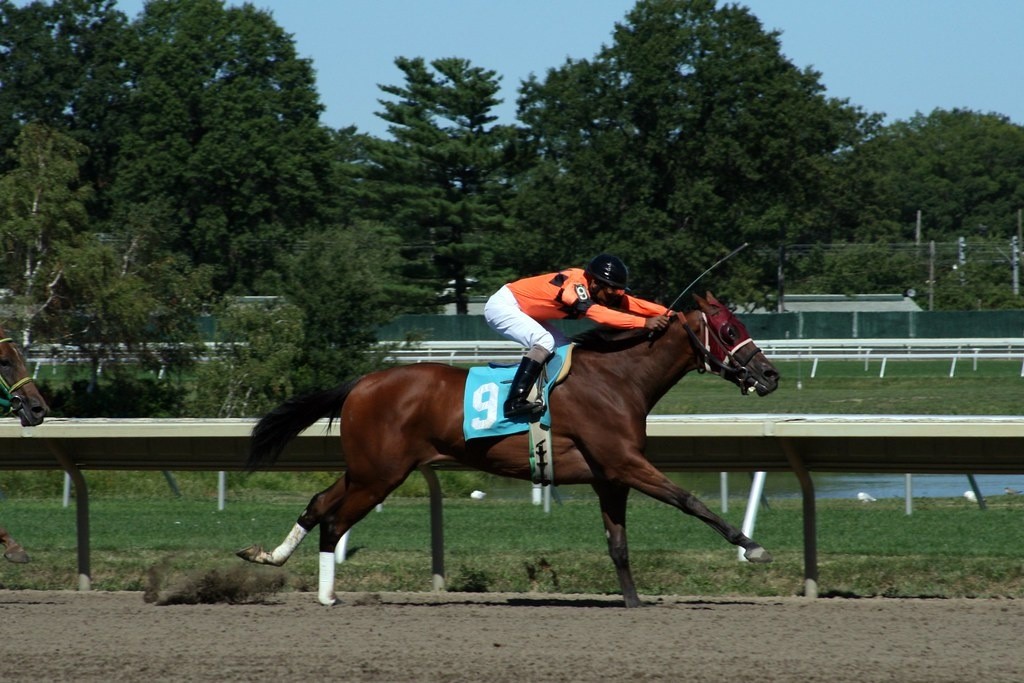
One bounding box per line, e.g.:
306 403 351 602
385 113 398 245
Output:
503 356 543 417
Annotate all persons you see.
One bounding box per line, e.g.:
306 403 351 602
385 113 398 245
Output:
484 254 673 418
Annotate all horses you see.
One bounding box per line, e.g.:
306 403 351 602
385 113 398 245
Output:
0 329 48 566
236 292 781 611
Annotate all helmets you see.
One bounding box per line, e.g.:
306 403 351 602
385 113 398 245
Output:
587 254 628 289
